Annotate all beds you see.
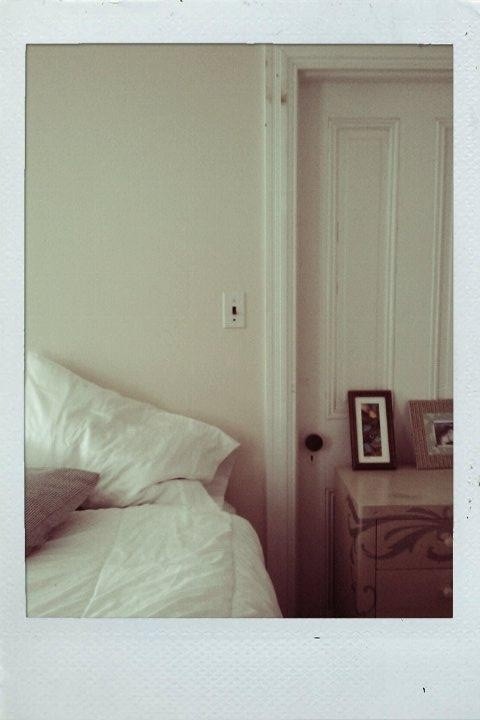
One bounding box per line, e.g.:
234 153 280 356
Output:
26 506 284 618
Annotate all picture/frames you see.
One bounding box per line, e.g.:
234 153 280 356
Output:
347 389 453 471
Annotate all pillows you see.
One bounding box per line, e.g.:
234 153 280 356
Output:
25 347 243 558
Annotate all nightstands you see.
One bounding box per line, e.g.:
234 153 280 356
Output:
332 465 453 617
